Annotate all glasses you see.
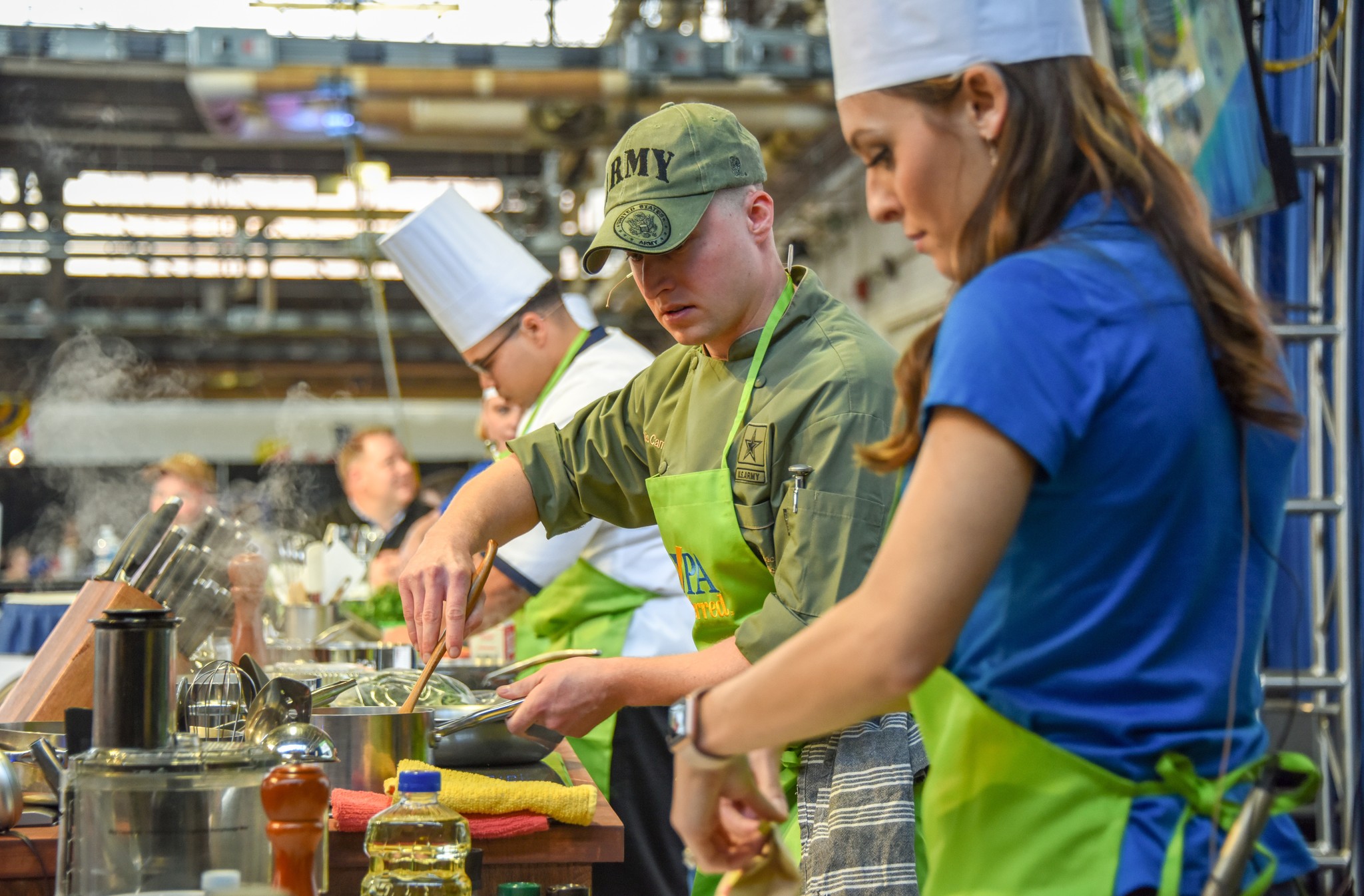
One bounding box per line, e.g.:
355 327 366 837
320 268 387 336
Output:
468 322 523 375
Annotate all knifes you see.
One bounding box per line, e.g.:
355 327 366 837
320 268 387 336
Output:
87 497 263 668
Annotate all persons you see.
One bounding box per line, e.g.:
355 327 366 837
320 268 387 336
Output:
402 382 527 561
670 0 1340 896
149 451 219 534
378 186 700 896
401 97 931 894
334 425 441 585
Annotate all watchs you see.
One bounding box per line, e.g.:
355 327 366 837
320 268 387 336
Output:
670 687 736 774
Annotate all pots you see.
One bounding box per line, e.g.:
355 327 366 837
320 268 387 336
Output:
0 714 81 808
280 683 528 799
419 644 599 687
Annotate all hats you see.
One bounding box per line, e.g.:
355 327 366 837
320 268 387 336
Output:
579 99 766 276
376 185 553 356
823 0 1094 99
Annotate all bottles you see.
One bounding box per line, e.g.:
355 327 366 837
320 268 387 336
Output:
359 771 476 896
91 606 184 755
